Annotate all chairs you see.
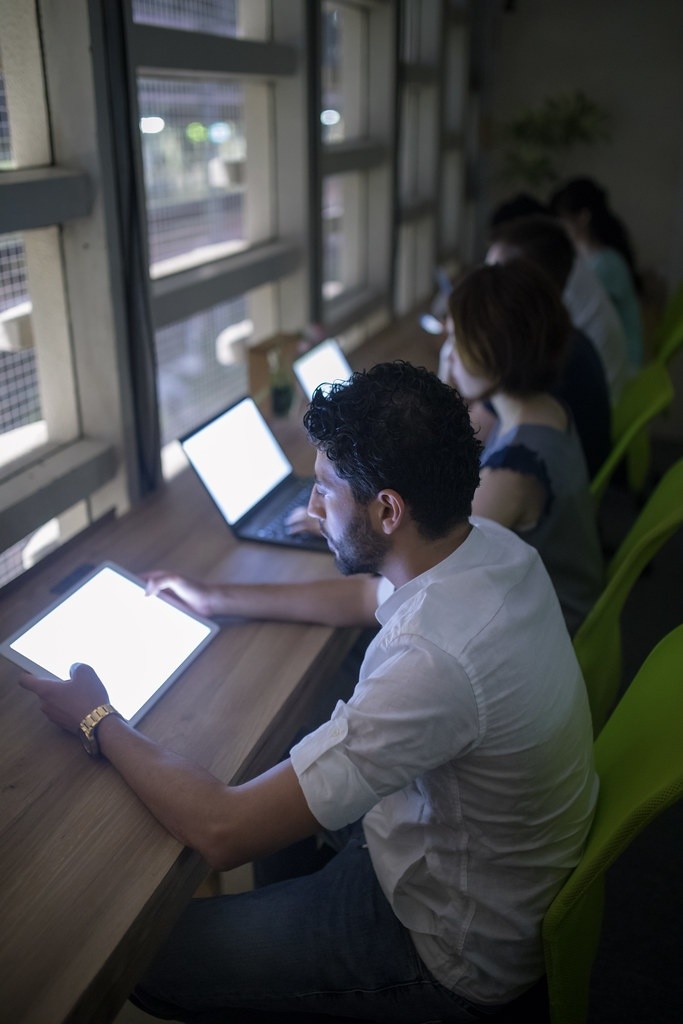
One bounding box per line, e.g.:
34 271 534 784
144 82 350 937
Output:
621 316 683 492
541 622 683 1024
571 462 683 740
588 363 675 503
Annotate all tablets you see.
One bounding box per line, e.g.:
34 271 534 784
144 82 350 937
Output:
0 560 221 730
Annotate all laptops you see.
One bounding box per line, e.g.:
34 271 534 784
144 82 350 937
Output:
175 395 334 552
293 337 353 401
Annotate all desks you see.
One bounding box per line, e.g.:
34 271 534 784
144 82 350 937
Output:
0 320 474 1024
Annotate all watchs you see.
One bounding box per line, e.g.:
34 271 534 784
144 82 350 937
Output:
80 703 125 759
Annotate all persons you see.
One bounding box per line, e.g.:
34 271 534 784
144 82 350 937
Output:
17 363 600 1024
283 180 648 631
